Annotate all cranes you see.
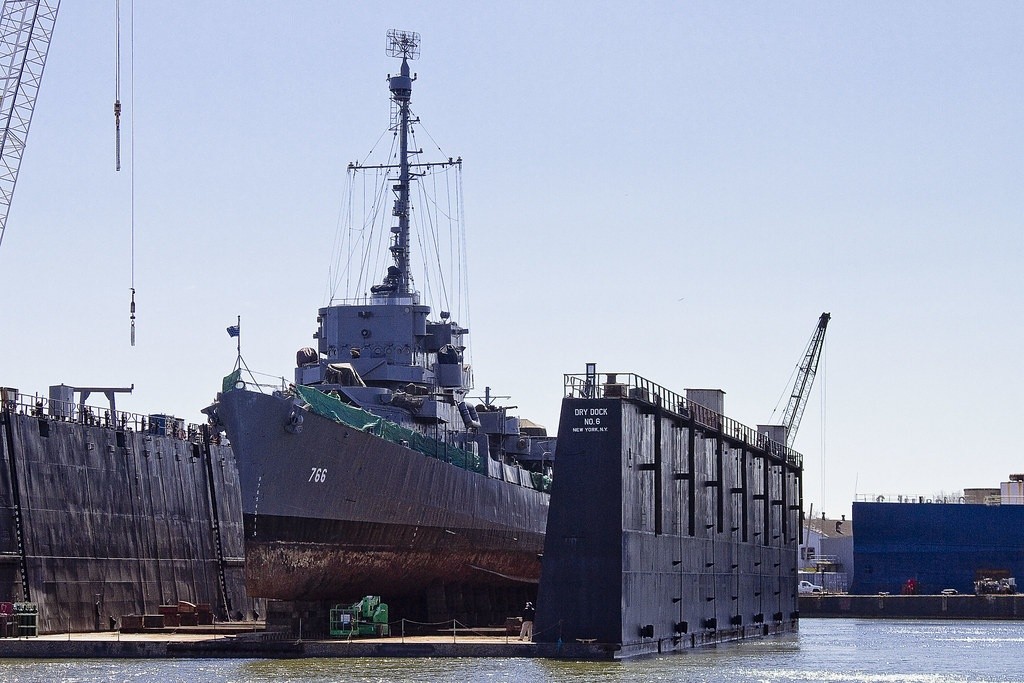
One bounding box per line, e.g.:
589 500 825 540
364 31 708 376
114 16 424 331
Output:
0 0 144 353
761 311 833 451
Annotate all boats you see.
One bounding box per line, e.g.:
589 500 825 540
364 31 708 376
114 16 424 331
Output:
200 27 559 596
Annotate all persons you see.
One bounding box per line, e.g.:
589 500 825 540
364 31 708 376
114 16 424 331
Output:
516 601 535 641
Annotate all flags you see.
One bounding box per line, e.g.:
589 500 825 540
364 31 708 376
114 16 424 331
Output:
228 324 240 337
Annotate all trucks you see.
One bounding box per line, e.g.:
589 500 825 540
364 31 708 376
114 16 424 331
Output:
798 581 822 593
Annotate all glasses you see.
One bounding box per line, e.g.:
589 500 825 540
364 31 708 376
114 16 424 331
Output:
526 604 529 605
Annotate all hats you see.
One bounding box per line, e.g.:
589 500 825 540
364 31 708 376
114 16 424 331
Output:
529 602 532 606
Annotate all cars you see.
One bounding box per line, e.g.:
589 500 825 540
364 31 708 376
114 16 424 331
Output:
941 588 959 595
